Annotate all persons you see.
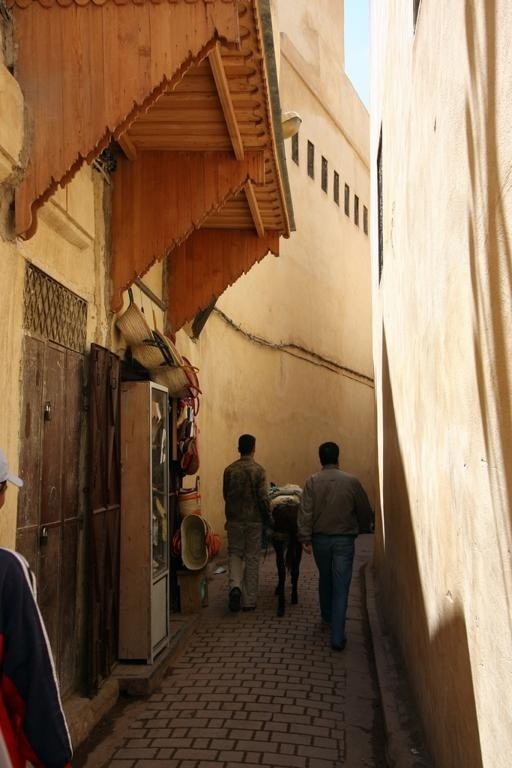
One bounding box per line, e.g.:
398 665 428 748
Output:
222 432 272 615
297 442 373 654
0 449 76 767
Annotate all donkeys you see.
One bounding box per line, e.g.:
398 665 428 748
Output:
263 482 303 617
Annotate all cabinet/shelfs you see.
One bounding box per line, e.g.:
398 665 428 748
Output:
121 381 173 666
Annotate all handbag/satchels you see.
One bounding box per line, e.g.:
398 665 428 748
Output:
176 474 202 518
133 327 184 370
115 303 153 346
151 355 199 398
180 513 211 570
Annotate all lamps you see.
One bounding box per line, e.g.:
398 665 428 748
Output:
281 109 303 138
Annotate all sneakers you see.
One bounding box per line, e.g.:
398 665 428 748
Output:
228 587 257 612
332 637 347 651
